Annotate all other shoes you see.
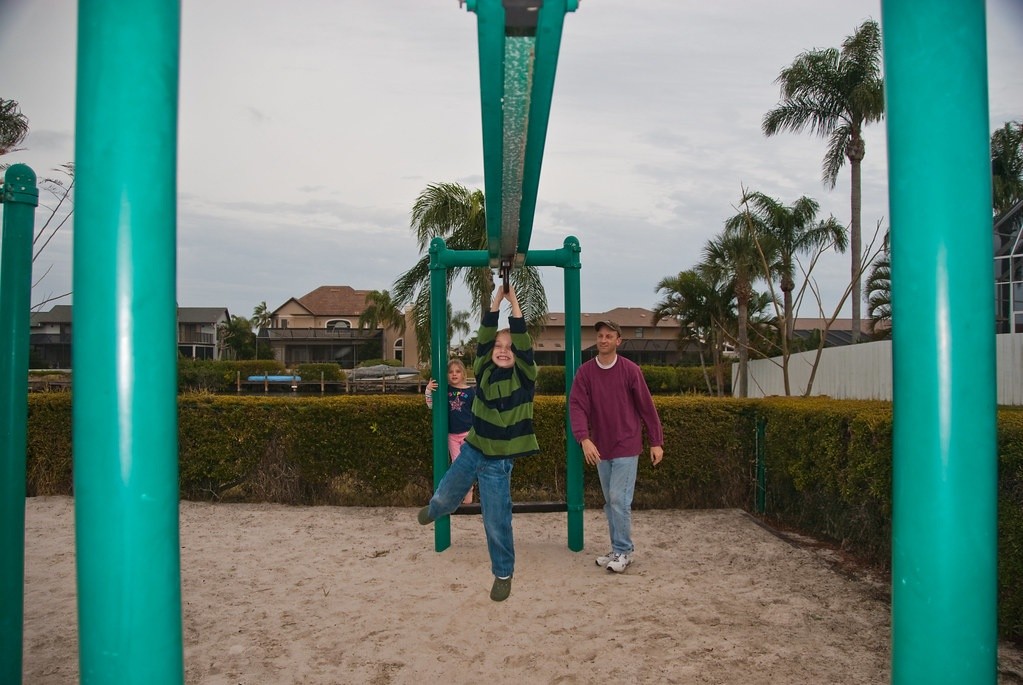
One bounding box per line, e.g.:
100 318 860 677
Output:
418 505 437 525
490 574 512 601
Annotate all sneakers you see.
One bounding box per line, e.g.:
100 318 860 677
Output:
606 552 632 572
596 551 614 566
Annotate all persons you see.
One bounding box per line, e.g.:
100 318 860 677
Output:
569 321 664 572
425 359 473 503
418 285 540 602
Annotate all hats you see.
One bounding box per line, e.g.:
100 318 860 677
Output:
594 320 622 337
